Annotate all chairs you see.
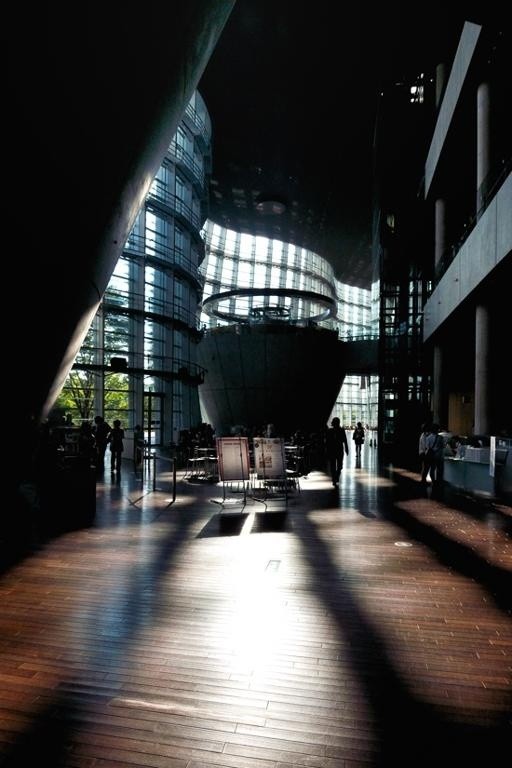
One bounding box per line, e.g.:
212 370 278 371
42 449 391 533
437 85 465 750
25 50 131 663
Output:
184 436 304 485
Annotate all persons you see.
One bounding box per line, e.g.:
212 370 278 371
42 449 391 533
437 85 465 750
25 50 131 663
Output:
418 422 444 490
325 418 348 483
65 414 124 472
352 421 365 459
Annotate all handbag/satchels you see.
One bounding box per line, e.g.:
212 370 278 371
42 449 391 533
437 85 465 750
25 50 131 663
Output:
426 448 436 465
